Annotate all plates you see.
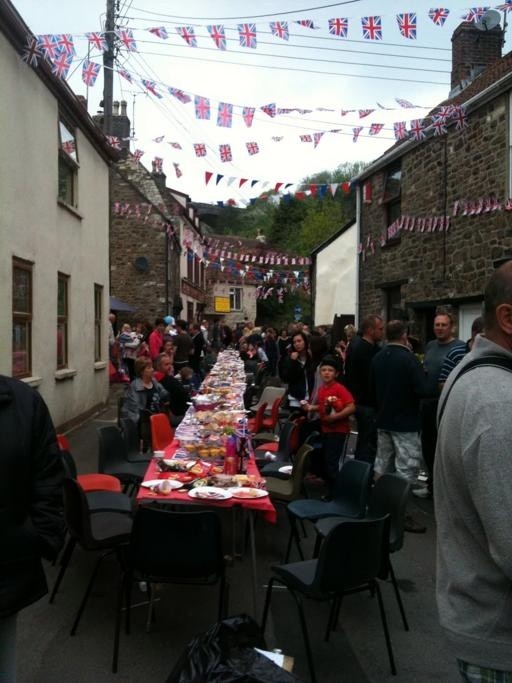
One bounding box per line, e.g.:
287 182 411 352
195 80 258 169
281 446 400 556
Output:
190 348 247 409
139 410 270 502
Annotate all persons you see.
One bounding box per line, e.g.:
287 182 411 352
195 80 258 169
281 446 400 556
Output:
0 374 68 683
431 259 512 683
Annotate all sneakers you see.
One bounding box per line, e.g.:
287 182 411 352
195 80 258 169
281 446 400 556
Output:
403 517 429 535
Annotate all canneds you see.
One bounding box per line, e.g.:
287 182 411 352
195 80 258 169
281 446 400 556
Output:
224 457 236 475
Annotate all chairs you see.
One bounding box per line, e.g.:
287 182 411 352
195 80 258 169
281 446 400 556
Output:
55 343 415 681
